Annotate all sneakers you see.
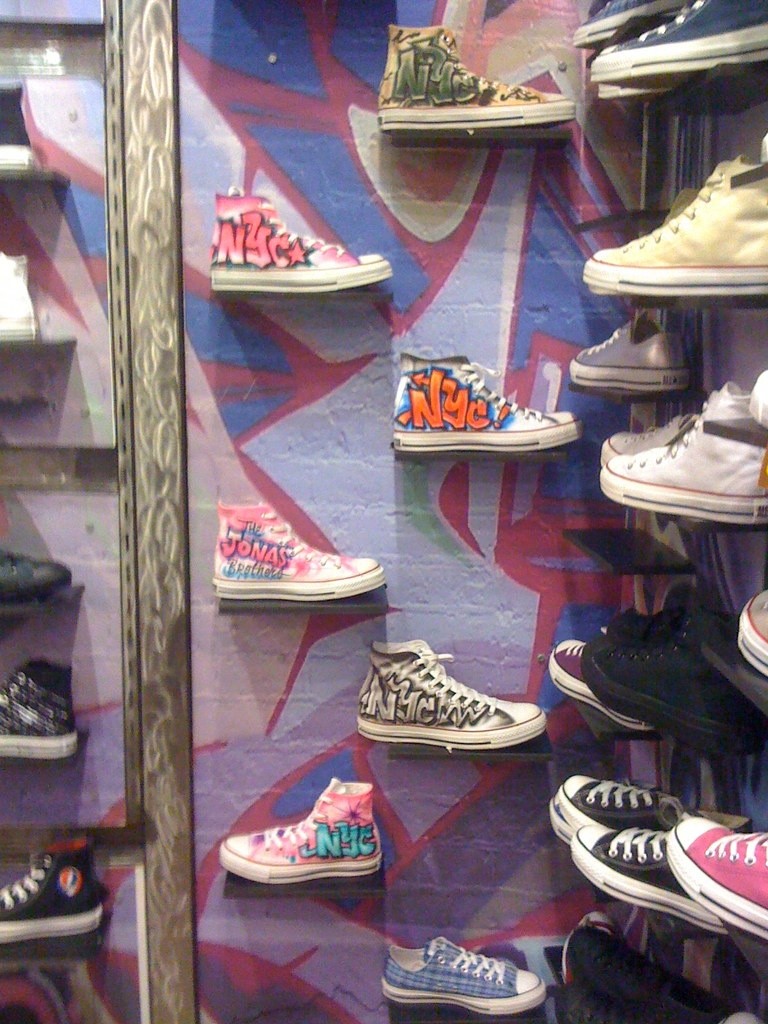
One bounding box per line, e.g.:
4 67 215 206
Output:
566 307 691 393
2 657 80 761
208 191 395 295
735 588 768 679
355 639 547 751
548 605 767 760
217 776 383 885
209 496 388 603
664 816 768 941
600 381 768 526
375 24 578 133
579 154 768 298
548 774 754 936
1 837 105 943
0 85 46 173
380 937 547 1016
1 248 40 341
0 548 75 617
391 351 585 453
571 0 768 99
553 909 748 1024
